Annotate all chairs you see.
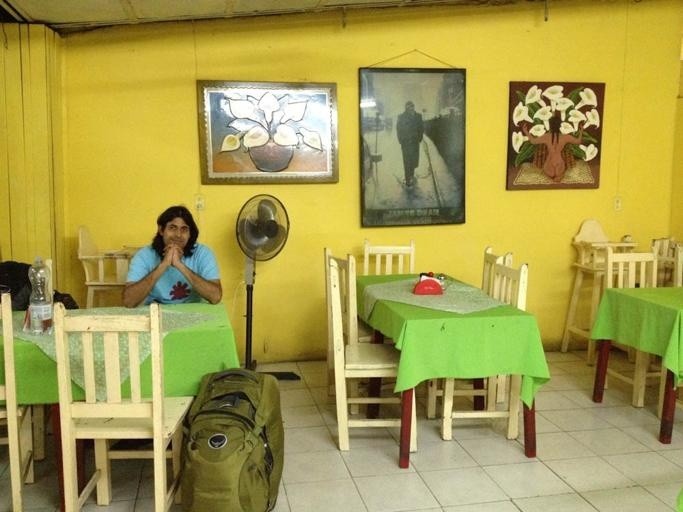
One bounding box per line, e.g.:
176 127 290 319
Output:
560 222 683 444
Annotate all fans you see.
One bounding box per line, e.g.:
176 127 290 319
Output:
234 195 301 382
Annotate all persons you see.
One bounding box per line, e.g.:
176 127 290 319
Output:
396 101 425 187
523 114 587 184
112 205 223 450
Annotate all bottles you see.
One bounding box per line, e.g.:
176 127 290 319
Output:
26 255 51 333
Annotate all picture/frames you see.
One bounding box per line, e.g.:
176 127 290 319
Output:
358 65 468 228
195 78 340 186
505 80 603 190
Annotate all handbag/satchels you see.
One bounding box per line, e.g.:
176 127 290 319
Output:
173 369 284 512
